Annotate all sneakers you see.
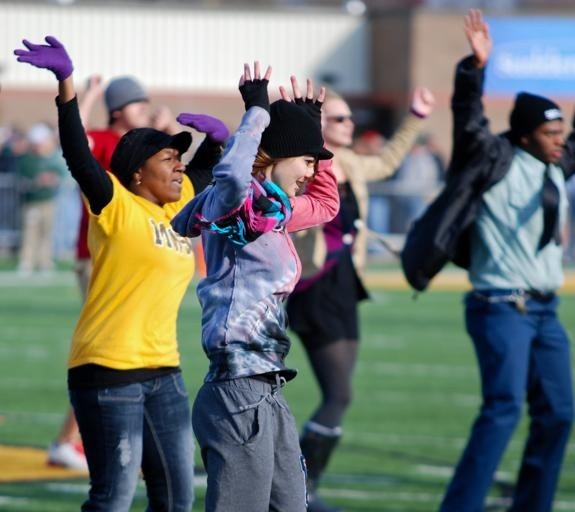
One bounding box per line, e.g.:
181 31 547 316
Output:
46 438 88 470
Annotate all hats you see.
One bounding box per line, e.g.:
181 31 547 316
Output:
109 128 193 188
509 91 564 139
260 98 334 161
104 77 149 113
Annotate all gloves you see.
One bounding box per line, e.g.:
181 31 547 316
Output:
288 98 324 110
13 35 75 81
175 113 229 143
238 78 270 111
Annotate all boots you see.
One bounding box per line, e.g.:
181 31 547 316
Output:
300 421 344 512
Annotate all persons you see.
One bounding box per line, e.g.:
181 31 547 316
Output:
167 57 343 512
45 72 178 471
399 5 573 512
356 133 444 254
0 123 80 276
12 35 230 511
286 87 437 512
